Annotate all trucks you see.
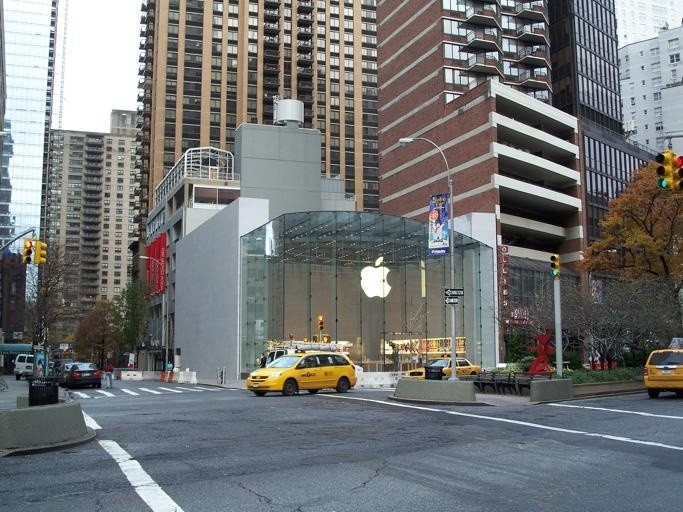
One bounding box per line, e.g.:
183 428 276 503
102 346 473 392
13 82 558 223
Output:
266 348 351 369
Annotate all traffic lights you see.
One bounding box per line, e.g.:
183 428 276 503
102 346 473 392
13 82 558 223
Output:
322 336 331 343
671 153 682 193
22 239 32 265
317 316 323 330
33 240 47 264
550 254 560 279
655 151 673 191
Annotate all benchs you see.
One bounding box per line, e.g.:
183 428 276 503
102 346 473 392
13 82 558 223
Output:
473 372 552 396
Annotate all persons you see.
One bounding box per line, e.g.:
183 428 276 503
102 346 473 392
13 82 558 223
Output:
104 358 114 389
165 361 173 383
258 353 266 368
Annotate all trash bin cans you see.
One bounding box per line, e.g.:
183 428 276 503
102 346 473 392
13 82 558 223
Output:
26 377 60 406
583 364 590 369
424 366 445 380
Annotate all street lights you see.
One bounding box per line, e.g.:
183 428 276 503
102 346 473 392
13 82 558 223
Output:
139 254 169 370
398 136 457 379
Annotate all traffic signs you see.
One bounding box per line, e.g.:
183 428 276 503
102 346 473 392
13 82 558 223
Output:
444 288 464 296
444 297 458 304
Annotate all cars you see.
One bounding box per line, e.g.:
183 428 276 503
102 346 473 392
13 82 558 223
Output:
11 353 102 388
246 350 357 395
409 357 480 380
643 349 682 398
